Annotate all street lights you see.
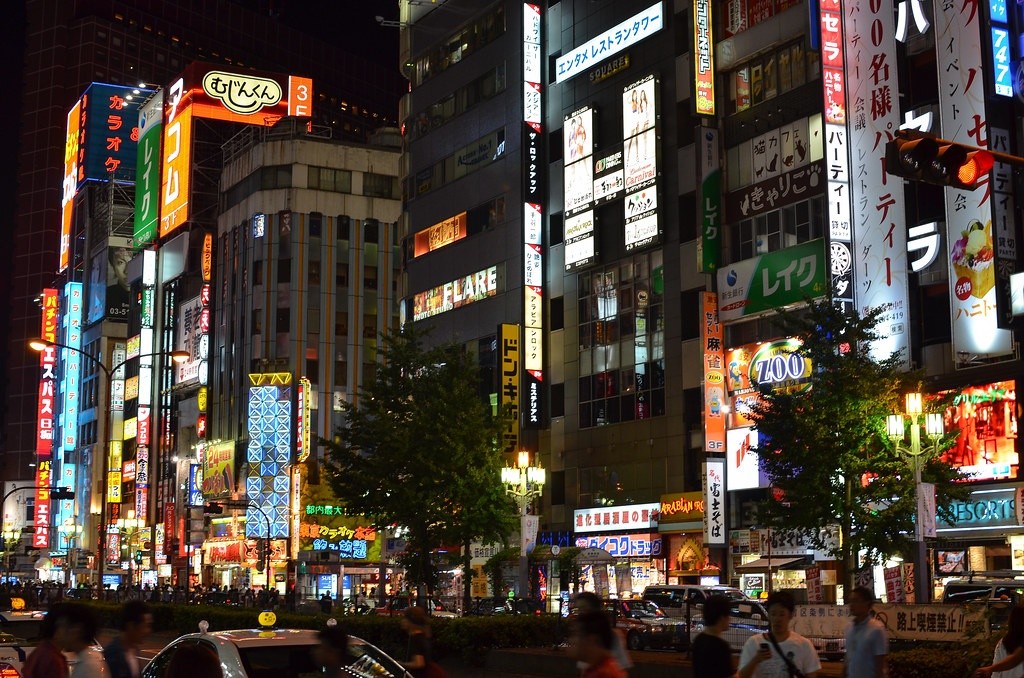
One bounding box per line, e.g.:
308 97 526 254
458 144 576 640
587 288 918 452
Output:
0 523 19 593
887 393 945 605
117 510 145 589
501 448 546 613
29 337 190 600
58 518 82 588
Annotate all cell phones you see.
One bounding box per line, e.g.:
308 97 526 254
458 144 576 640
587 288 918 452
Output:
759 643 772 659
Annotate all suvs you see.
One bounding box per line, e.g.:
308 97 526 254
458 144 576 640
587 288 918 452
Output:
565 598 687 650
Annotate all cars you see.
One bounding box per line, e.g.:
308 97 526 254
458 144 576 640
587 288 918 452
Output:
0 610 107 678
138 628 414 678
376 597 458 623
461 597 542 616
805 636 848 661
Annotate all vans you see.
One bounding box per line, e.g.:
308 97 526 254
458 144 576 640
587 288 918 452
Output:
940 579 1024 630
643 585 770 652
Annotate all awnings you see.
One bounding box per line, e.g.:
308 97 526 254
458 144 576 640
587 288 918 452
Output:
735 557 807 574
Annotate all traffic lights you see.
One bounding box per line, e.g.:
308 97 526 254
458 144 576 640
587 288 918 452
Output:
884 135 995 192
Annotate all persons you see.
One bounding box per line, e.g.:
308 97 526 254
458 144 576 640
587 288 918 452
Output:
690 595 734 678
107 246 137 317
311 626 352 678
354 586 415 614
973 588 1024 678
569 592 634 678
0 580 302 614
839 586 889 678
21 600 153 678
737 596 822 678
395 607 433 678
320 590 332 613
